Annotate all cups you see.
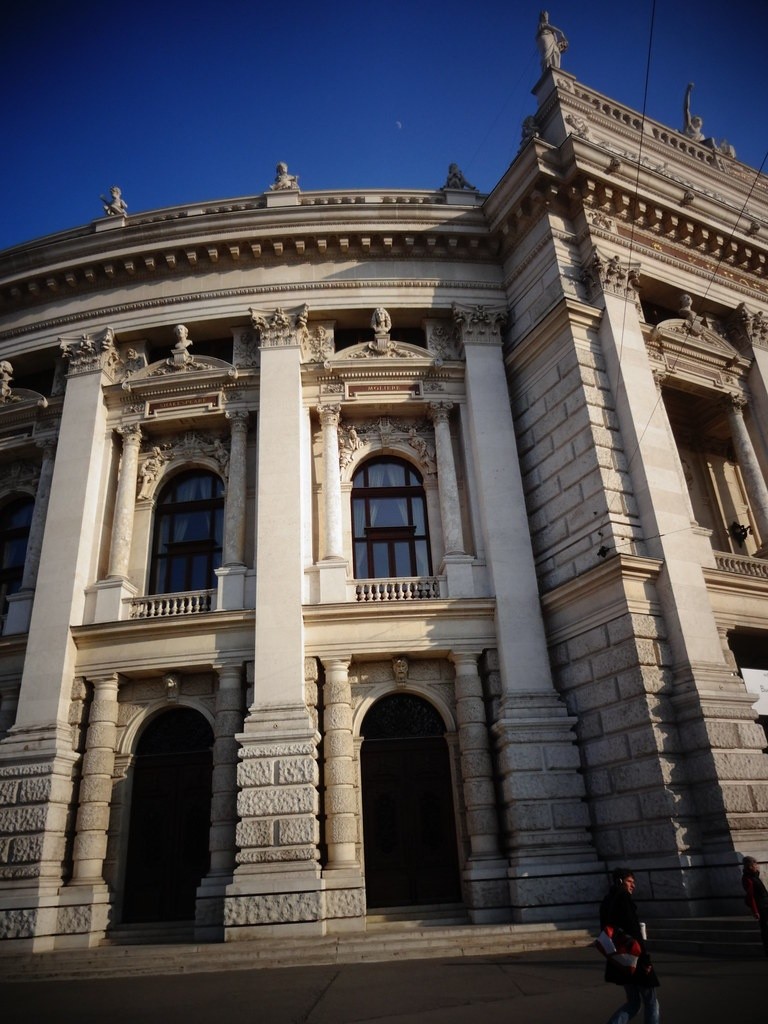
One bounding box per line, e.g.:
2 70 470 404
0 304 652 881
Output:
639 923 647 940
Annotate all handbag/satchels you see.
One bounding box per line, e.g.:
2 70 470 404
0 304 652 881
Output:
594 926 641 986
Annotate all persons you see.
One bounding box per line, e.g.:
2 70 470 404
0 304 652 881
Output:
165 675 184 703
269 162 297 191
447 161 475 191
174 325 193 348
104 186 127 215
536 8 569 72
684 81 720 151
392 657 412 686
678 293 697 321
742 857 768 957
137 436 231 499
339 428 432 475
599 867 661 1024
0 361 15 387
369 308 390 333
